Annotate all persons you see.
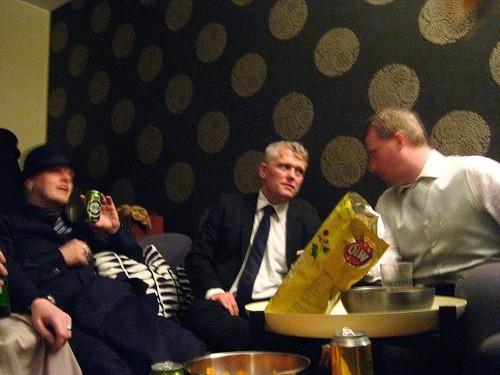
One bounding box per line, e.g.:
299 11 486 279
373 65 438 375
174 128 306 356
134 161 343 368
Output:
0 128 210 375
179 139 333 375
360 108 500 375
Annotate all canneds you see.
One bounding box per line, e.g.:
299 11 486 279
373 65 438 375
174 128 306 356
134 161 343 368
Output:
150 360 185 375
331 327 373 375
83 190 102 222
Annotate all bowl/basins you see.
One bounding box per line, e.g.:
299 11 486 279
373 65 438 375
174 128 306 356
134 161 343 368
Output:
340 285 436 314
184 351 311 375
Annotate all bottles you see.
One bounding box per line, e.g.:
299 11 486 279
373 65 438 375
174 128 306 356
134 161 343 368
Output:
0 262 11 318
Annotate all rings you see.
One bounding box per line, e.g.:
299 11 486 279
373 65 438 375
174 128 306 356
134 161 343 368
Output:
67 326 72 330
83 245 88 256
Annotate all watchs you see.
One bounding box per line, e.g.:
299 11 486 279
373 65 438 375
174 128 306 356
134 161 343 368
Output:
45 294 55 304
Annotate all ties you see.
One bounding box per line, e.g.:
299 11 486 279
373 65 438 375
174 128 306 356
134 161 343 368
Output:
235 205 273 315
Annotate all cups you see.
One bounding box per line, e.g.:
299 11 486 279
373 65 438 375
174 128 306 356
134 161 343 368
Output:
380 262 413 286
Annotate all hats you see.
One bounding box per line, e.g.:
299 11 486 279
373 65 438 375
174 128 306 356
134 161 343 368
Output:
20 141 77 181
